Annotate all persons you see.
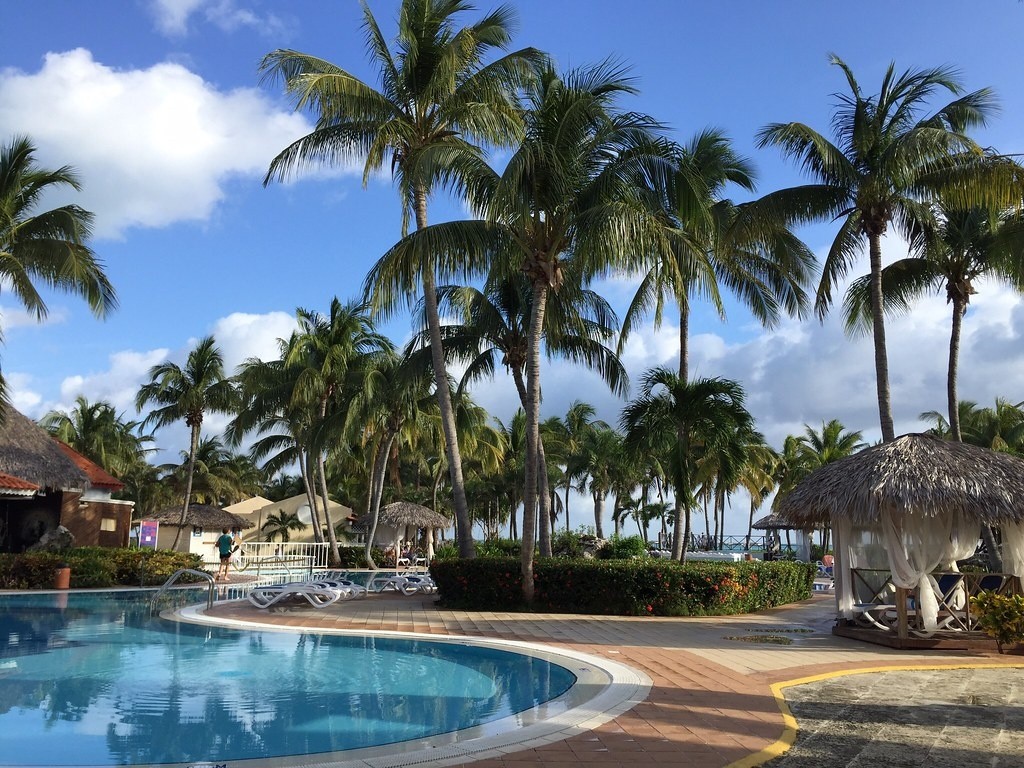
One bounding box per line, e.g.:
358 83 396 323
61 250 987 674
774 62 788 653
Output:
403 541 414 560
216 529 233 578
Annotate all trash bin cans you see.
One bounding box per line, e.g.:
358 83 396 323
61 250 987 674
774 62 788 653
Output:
55 562 71 589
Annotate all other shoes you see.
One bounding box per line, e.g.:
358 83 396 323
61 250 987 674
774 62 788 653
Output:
225 576 230 580
216 574 220 580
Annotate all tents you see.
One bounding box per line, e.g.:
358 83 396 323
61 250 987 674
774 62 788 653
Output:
770 434 1024 637
752 513 831 555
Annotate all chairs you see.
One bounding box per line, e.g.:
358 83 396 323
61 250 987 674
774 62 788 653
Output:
248 557 436 608
879 573 1008 637
853 571 965 631
792 558 835 581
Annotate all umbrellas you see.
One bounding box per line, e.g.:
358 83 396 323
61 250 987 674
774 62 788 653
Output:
0 396 91 493
356 500 451 545
